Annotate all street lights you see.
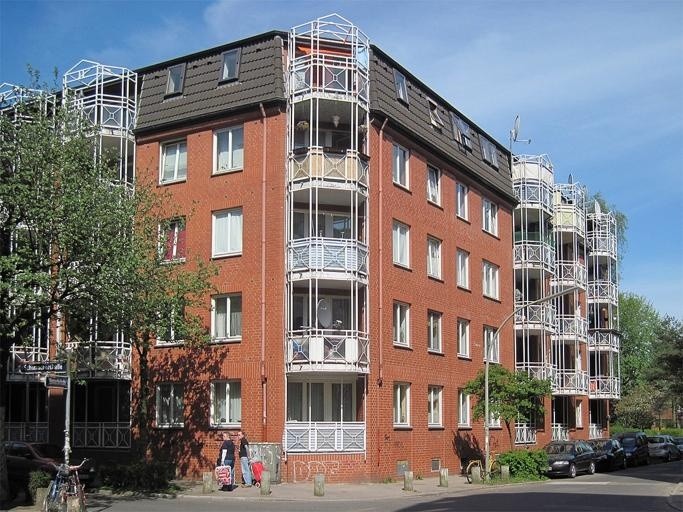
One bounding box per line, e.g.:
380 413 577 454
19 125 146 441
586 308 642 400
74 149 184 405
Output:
484 284 585 484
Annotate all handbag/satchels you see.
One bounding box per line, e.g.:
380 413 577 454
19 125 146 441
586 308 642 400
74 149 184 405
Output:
217 457 225 466
215 463 231 486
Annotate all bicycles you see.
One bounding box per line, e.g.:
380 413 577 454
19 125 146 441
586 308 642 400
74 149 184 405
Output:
467 450 501 484
42 458 90 512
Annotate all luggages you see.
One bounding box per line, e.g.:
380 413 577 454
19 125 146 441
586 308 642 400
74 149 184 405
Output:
249 459 265 488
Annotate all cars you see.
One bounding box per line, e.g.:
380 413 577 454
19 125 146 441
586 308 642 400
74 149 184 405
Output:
3 440 96 486
543 431 683 478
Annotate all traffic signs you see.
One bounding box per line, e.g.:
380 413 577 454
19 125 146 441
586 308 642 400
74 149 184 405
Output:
19 360 68 390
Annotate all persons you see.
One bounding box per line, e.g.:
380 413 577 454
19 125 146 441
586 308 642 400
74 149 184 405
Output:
219 432 235 491
238 431 252 488
